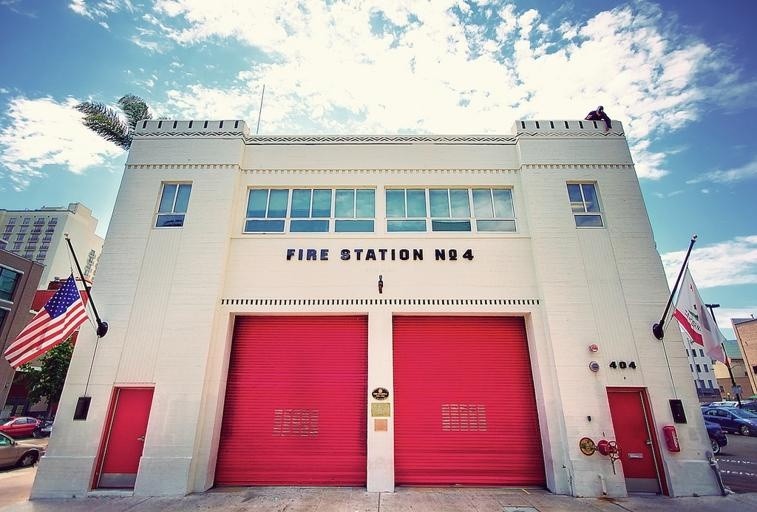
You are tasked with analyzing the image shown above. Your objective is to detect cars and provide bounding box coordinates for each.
[33,420,53,438]
[0,415,43,438]
[0,432,44,471]
[703,400,757,436]
[705,420,727,454]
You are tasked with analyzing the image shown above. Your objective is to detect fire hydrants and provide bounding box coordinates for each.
[580,437,623,460]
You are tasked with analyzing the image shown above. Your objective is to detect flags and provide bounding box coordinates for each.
[668,264,727,366]
[3,271,89,370]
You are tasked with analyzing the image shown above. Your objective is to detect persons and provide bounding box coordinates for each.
[584,106,611,131]
